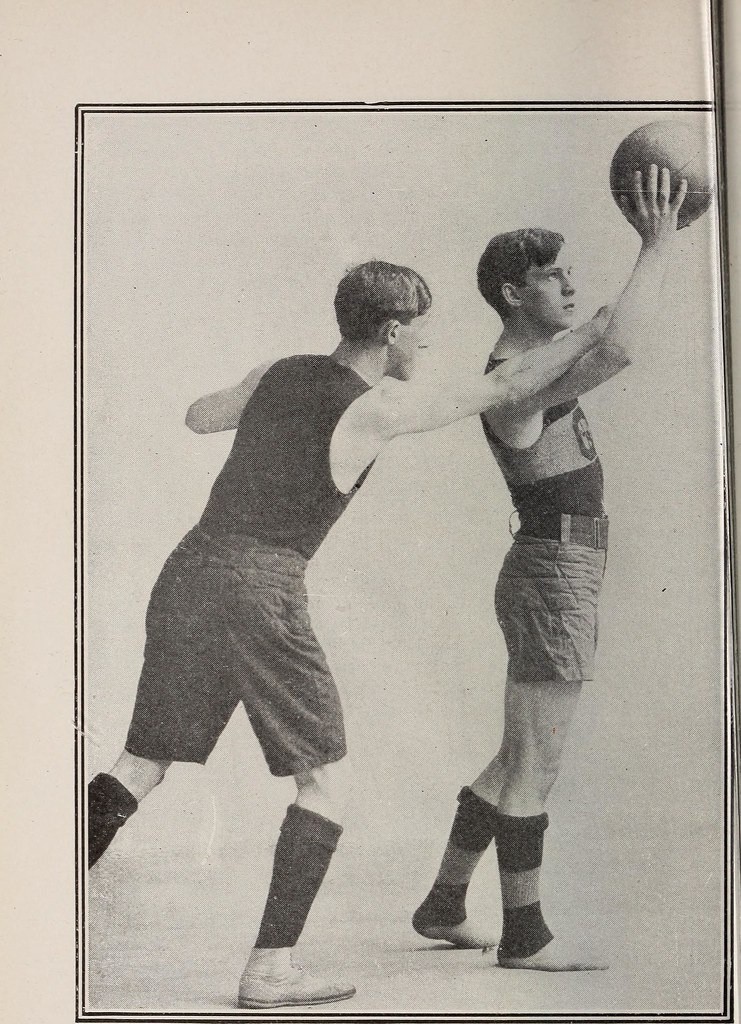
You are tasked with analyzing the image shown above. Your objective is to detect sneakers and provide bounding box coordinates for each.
[239,953,356,1009]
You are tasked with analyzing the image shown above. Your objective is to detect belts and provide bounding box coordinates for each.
[514,512,609,549]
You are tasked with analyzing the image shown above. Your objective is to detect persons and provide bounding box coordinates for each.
[412,164,688,974]
[85,261,618,1007]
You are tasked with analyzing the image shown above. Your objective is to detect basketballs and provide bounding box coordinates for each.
[610,118,712,229]
